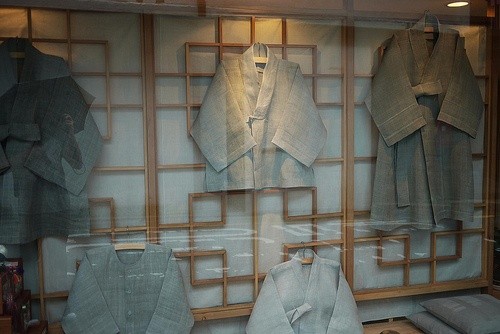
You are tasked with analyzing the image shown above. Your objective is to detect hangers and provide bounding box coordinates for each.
[114,225,145,250]
[8,35,25,59]
[424,8,436,33]
[253,41,267,63]
[299,240,314,265]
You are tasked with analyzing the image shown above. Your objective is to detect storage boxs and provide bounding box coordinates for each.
[0,315,14,334]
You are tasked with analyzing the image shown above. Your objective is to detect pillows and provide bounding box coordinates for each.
[405,310,462,334]
[422,293,500,334]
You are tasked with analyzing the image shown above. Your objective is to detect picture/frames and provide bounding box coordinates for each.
[10,288,33,334]
[6,257,24,291]
[25,320,49,334]
